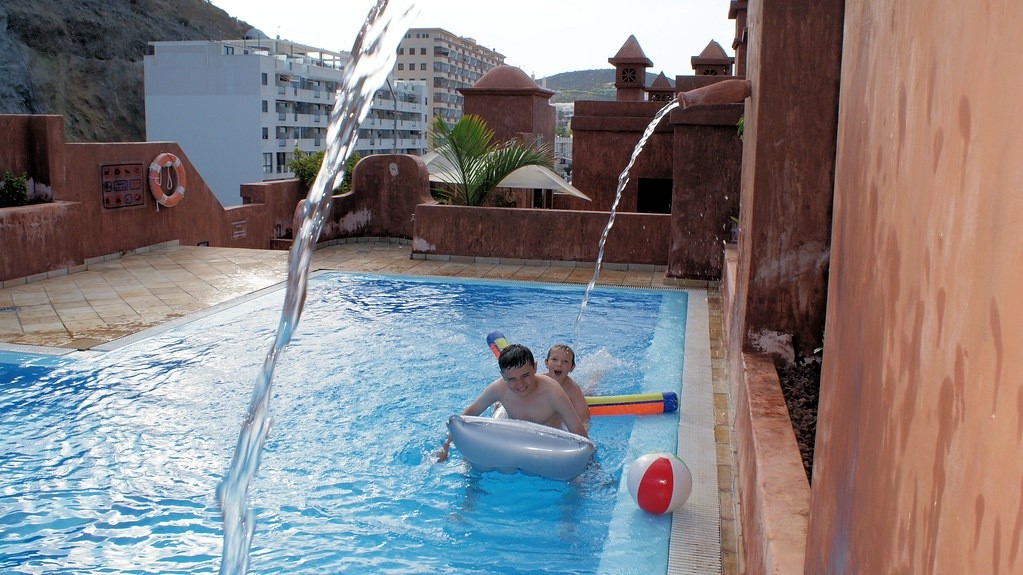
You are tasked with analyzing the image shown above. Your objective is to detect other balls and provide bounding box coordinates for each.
[627,449,693,516]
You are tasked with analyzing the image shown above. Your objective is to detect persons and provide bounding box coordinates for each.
[435,343,590,462]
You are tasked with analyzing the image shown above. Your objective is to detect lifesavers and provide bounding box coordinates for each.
[149,152,187,209]
[486,332,679,414]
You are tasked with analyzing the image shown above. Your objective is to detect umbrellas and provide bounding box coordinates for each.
[419,142,593,206]
